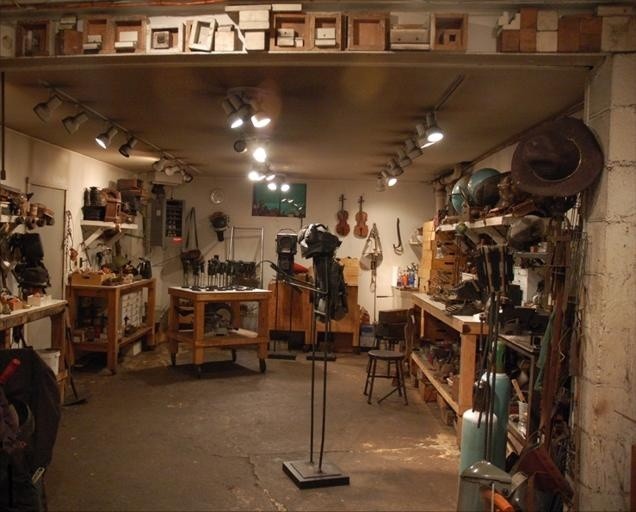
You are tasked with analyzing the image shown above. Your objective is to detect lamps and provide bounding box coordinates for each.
[33,79,202,182]
[223,94,290,192]
[377,73,465,192]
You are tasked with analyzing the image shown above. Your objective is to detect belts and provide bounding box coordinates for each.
[549,215,569,447]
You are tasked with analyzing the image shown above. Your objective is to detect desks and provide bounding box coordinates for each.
[66,279,156,374]
[169,286,272,378]
[412,292,489,446]
[0,300,68,410]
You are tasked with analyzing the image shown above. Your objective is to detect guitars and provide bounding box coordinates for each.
[354,196,368,237]
[336,195,349,236]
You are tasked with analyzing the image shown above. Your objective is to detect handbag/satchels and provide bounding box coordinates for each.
[180,249,205,265]
[0,348,61,474]
[509,443,573,503]
[361,253,383,269]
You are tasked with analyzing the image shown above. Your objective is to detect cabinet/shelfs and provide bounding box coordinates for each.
[392,287,416,310]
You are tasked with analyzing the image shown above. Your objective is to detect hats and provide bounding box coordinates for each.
[511,118,603,197]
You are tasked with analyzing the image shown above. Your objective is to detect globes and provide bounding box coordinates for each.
[447,168,501,216]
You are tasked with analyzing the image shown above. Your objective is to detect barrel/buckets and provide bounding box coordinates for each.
[37,347,61,376]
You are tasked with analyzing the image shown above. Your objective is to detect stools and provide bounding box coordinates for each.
[363,350,408,405]
